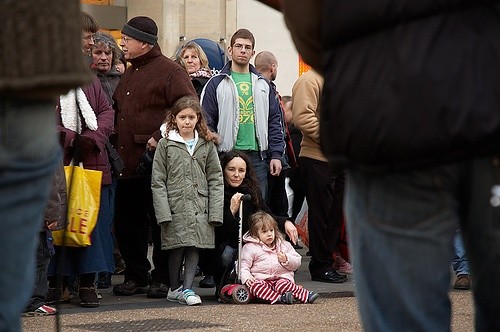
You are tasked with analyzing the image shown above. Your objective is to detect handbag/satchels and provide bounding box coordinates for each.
[108,146,127,175]
[51,158,103,247]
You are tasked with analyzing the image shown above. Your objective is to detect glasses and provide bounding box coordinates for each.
[121,35,133,40]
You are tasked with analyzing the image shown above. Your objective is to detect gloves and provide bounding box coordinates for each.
[72,132,100,153]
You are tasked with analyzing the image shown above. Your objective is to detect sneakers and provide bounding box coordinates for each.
[113,280,150,296]
[166,284,183,303]
[178,288,202,306]
[148,282,170,299]
[20,304,56,317]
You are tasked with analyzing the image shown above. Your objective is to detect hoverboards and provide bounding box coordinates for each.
[215,193,256,306]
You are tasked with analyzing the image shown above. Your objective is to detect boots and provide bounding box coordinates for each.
[47,280,62,304]
[79,279,99,308]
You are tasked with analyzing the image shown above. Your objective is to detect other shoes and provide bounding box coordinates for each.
[339,262,353,274]
[199,275,214,288]
[308,291,319,304]
[215,290,231,304]
[311,270,347,283]
[281,292,293,304]
[453,275,471,290]
[114,263,127,275]
[98,274,111,289]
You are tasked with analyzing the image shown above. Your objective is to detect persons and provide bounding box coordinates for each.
[0,0,90,332]
[258,1,500,332]
[171,41,214,288]
[150,97,224,306]
[200,27,285,197]
[54,14,125,308]
[239,210,319,307]
[254,52,353,282]
[451,234,471,290]
[110,15,198,299]
[211,151,270,294]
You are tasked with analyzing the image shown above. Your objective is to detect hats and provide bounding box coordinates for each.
[121,15,158,45]
